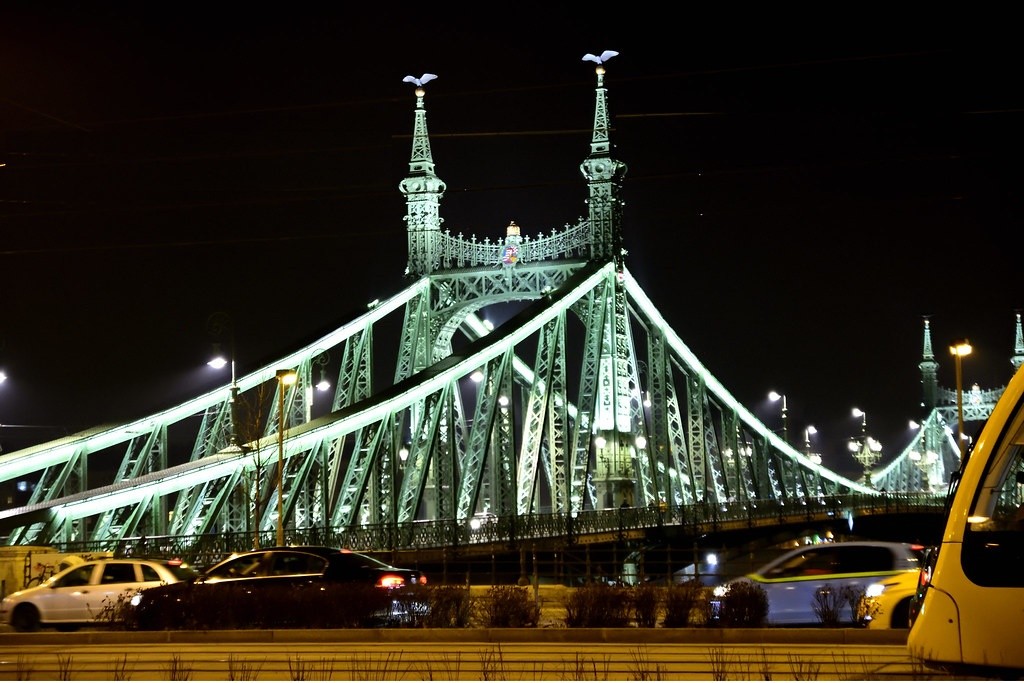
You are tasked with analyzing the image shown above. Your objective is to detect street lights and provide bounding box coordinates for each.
[805,425,817,460]
[275,369,299,548]
[846,408,882,489]
[208,356,242,445]
[399,444,408,477]
[949,342,973,462]
[768,391,788,442]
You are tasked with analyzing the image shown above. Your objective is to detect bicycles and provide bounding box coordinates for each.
[25,562,56,589]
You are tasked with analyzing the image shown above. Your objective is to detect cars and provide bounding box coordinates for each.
[907,363,1024,671]
[0,558,199,632]
[129,545,427,628]
[146,559,201,583]
[710,540,928,630]
